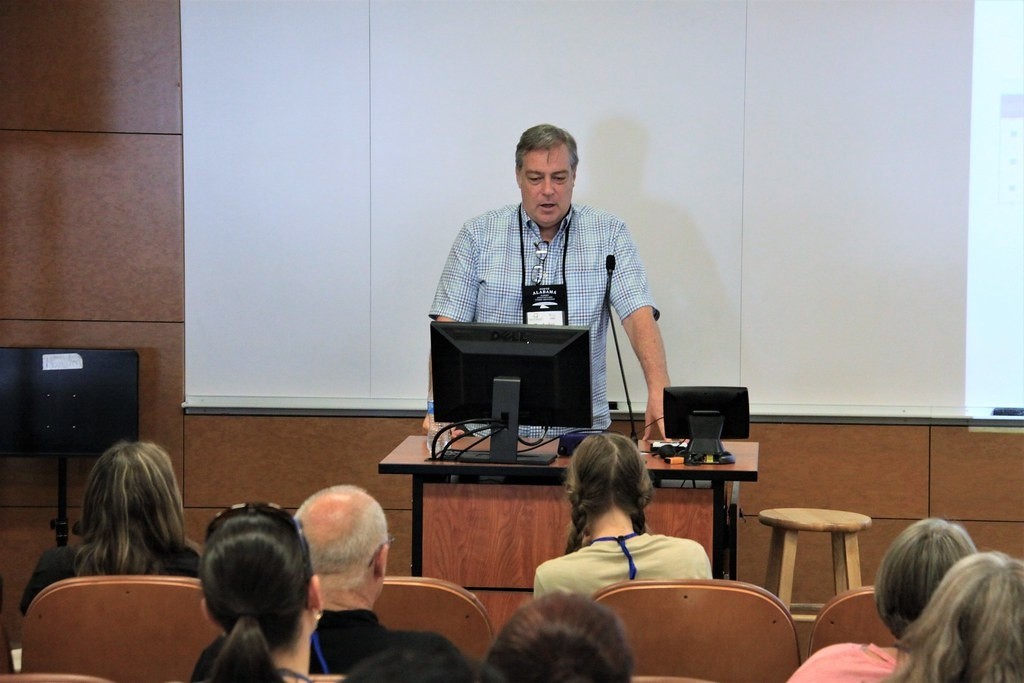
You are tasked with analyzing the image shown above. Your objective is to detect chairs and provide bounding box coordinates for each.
[376,577,491,662]
[21,576,219,683]
[809,588,892,659]
[587,579,801,683]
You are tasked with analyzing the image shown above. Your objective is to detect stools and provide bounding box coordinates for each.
[758,508,871,626]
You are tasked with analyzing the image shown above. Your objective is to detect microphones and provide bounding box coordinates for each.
[604,254,640,453]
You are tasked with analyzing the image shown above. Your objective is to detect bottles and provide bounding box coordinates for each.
[427,387,451,453]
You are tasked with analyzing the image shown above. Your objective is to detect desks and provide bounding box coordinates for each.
[377,435,760,624]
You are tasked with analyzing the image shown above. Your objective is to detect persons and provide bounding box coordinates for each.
[424,123,688,444]
[21,441,200,616]
[479,589,634,683]
[786,518,976,683]
[533,434,713,600]
[294,484,416,673]
[885,551,1024,683]
[339,634,472,683]
[191,504,344,683]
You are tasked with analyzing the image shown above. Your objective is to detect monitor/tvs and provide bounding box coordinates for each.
[430,321,594,466]
[664,387,750,464]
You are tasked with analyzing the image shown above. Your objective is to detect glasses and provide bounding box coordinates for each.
[530,240,549,288]
[204,501,300,542]
